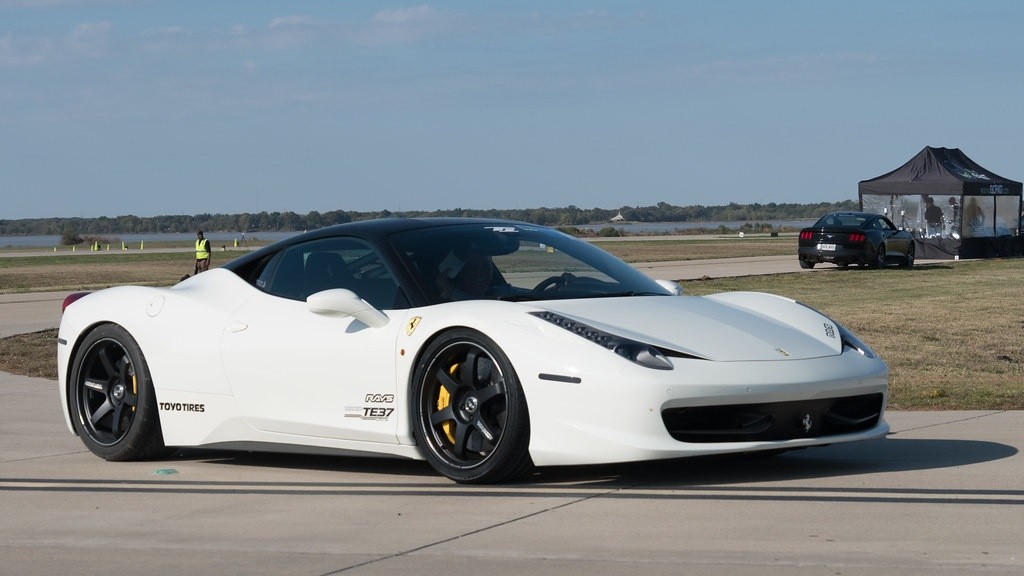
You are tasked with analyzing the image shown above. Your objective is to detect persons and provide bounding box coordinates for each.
[925,197,946,238]
[948,197,960,235]
[964,197,985,237]
[453,247,572,300]
[194,230,211,275]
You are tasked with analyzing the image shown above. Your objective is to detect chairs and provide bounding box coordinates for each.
[306,253,363,303]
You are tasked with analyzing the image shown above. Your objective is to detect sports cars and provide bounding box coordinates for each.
[56,216,890,484]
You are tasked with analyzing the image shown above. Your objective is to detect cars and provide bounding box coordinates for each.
[798,212,915,270]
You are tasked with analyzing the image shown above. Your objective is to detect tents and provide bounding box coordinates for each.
[858,145,1024,259]
